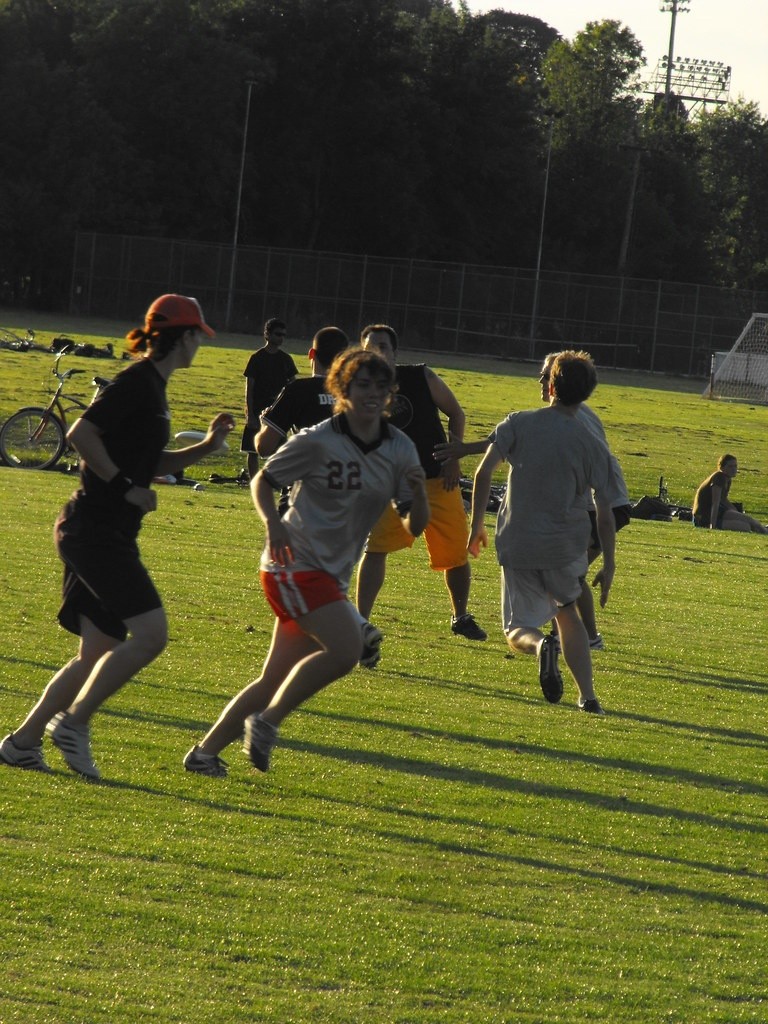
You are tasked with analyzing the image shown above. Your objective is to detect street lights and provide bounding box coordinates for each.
[614,142,659,270]
[527,104,567,358]
[223,72,260,329]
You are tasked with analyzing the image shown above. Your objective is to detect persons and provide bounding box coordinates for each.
[0,294,237,776]
[240,319,299,479]
[360,324,487,641]
[253,326,382,669]
[183,346,430,778]
[466,349,620,717]
[432,354,631,646]
[692,454,768,533]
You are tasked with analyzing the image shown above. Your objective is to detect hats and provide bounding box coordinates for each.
[145,294,216,337]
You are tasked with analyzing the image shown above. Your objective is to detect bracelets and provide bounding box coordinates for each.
[111,471,136,495]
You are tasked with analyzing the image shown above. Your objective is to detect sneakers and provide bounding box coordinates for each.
[0,733,52,772]
[45,711,100,778]
[549,631,562,650]
[589,632,603,649]
[360,622,382,669]
[244,713,277,773]
[578,697,606,715]
[450,615,488,641]
[183,745,229,778]
[538,636,563,703]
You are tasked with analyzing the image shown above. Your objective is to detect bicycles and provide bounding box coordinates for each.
[0,344,114,470]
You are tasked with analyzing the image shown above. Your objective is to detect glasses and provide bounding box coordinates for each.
[540,372,550,381]
[270,332,285,337]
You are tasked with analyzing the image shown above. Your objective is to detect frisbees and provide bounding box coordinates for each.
[174,432,229,455]
